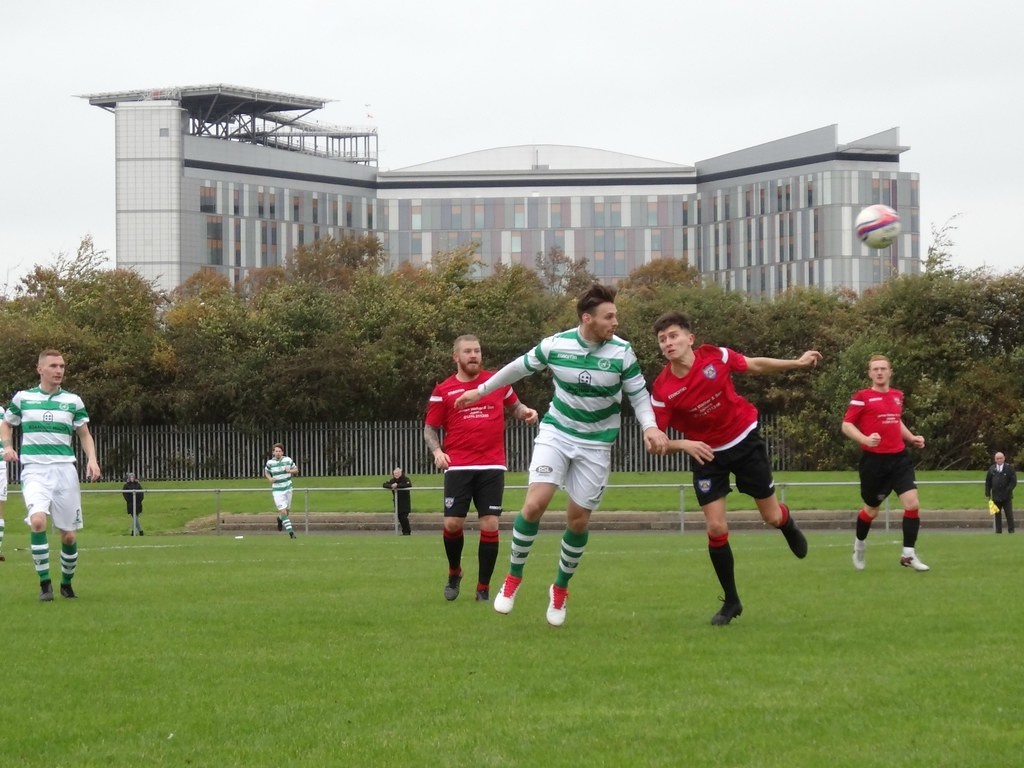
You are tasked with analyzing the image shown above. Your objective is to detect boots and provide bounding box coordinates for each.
[139,530,143,537]
[131,530,134,536]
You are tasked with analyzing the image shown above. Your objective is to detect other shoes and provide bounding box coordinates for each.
[289,531,296,538]
[0,554,5,561]
[277,517,284,531]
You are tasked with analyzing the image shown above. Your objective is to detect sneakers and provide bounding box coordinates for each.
[899,553,929,571]
[39,578,54,601]
[781,517,808,557]
[546,583,570,626]
[853,540,866,570]
[475,586,488,600]
[494,574,523,614]
[712,595,742,625]
[60,584,77,598]
[444,567,464,601]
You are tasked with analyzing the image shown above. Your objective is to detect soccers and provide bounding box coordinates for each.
[854,204,904,250]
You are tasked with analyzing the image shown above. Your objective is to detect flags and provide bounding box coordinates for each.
[988,498,1000,517]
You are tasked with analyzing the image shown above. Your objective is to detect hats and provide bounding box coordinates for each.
[127,473,135,482]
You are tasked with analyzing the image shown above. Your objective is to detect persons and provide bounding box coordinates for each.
[384,467,413,535]
[985,451,1018,534]
[841,354,931,570]
[122,473,145,535]
[264,443,299,539]
[642,313,824,627]
[0,350,100,601]
[454,289,669,625]
[423,333,539,601]
[0,406,12,565]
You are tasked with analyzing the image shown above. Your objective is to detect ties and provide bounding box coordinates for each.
[998,464,1001,472]
